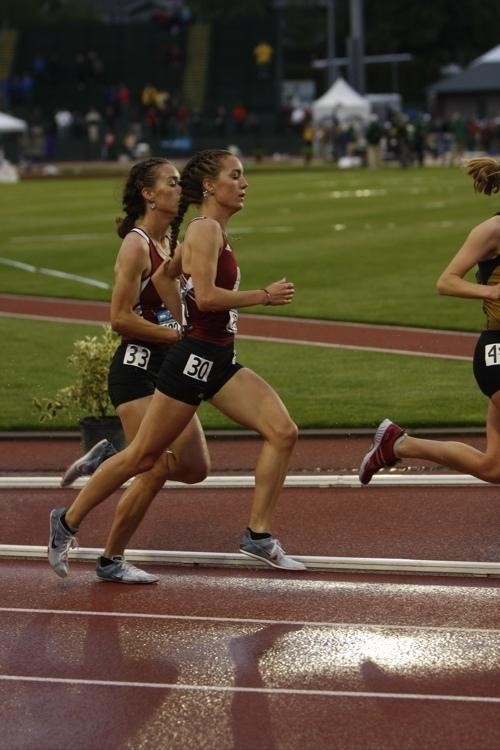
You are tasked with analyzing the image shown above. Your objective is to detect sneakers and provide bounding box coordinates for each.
[238,531,307,572]
[96,555,159,584]
[61,440,117,488]
[358,417,404,485]
[47,508,76,578]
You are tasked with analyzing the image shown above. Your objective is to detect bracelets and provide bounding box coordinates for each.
[261,285,273,308]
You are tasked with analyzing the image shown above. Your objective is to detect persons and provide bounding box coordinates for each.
[56,158,213,585]
[48,150,309,578]
[22,82,499,176]
[358,157,499,488]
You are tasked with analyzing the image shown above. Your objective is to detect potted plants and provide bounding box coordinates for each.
[29,324,122,453]
